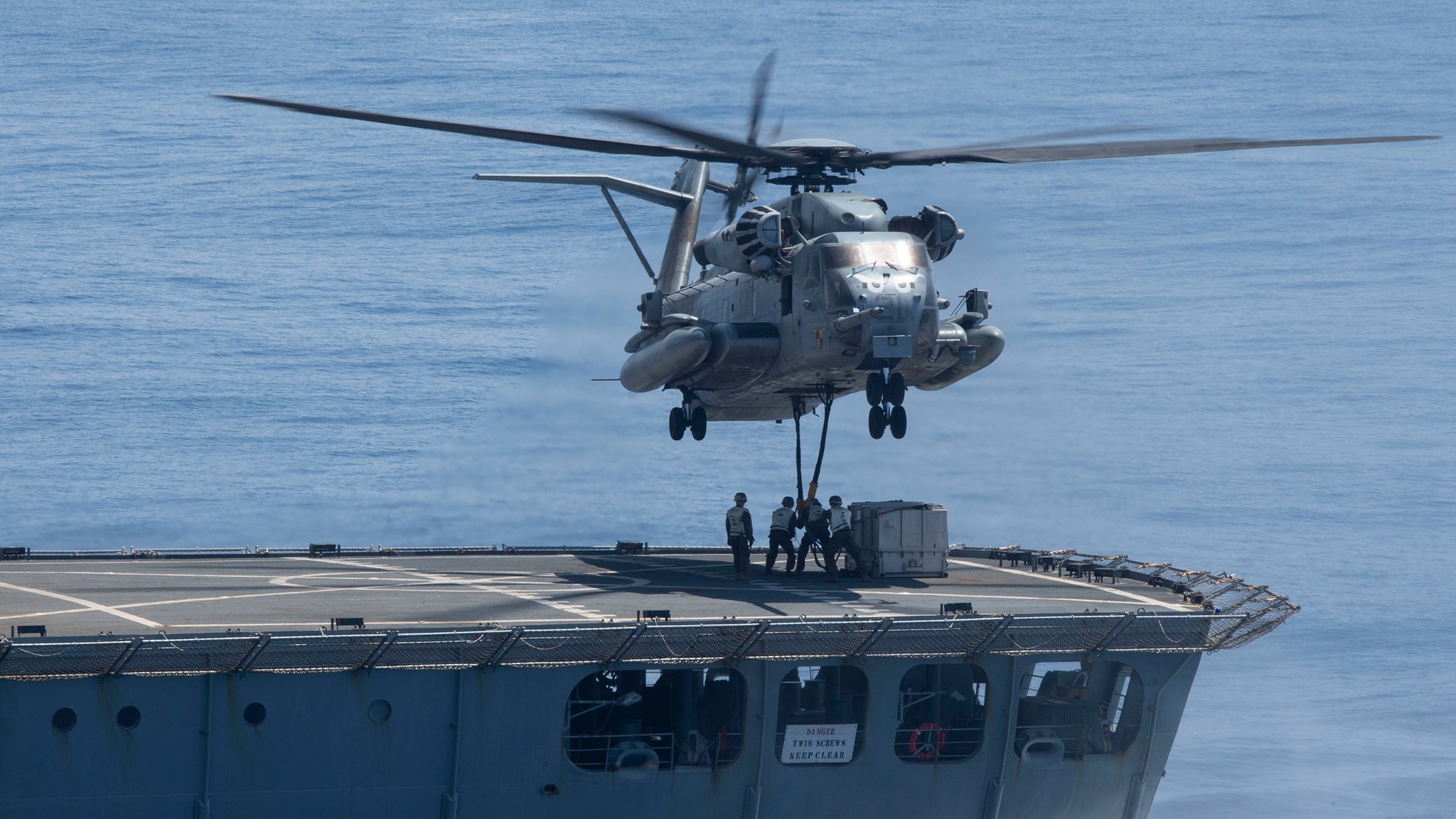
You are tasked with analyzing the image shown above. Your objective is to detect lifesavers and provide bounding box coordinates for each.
[909,722,946,761]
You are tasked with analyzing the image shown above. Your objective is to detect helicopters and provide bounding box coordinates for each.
[212,48,1440,443]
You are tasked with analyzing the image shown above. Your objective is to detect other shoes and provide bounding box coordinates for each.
[736,572,741,581]
[824,573,839,582]
[764,568,775,575]
[786,569,797,577]
[740,572,747,581]
[792,568,802,575]
[860,571,874,582]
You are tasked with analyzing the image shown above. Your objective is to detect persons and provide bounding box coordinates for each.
[794,497,838,577]
[819,496,873,583]
[764,497,797,576]
[726,492,755,582]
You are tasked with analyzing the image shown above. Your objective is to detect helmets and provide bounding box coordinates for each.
[781,497,794,505]
[734,492,747,501]
[812,498,822,506]
[829,496,842,505]
